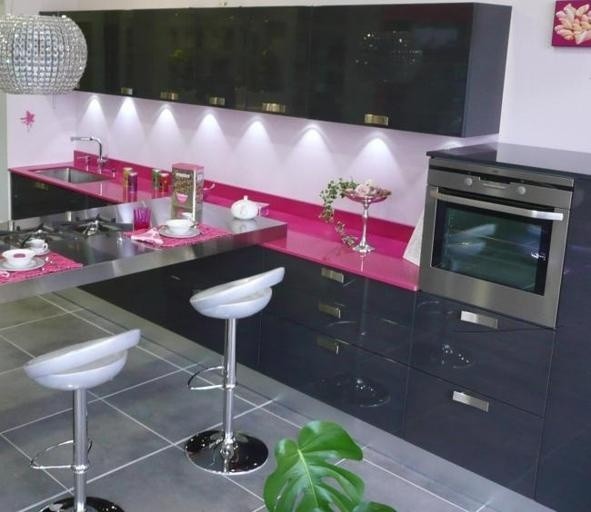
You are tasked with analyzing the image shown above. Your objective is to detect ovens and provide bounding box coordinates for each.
[420,157,574,332]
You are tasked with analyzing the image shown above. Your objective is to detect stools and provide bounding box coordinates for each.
[23,329,141,512]
[184,267,286,476]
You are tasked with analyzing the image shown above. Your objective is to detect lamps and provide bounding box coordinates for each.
[1,12,88,96]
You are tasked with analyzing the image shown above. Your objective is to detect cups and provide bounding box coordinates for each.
[31,239,48,254]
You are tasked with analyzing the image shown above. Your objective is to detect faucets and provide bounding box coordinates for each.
[69,136,103,160]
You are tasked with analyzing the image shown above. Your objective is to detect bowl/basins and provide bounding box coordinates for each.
[166,219,194,234]
[1,249,36,267]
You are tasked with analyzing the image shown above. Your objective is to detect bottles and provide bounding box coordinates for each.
[160,173,168,192]
[123,167,133,185]
[152,169,161,188]
[128,172,138,191]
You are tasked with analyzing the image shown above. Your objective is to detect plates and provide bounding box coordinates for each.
[28,247,49,256]
[159,227,201,238]
[0,256,45,272]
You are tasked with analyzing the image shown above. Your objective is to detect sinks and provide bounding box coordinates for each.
[29,166,112,185]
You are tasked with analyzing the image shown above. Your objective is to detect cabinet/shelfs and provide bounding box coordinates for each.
[133,9,181,101]
[12,173,108,218]
[78,245,254,369]
[340,3,512,137]
[256,248,556,497]
[40,11,89,93]
[89,11,133,97]
[239,7,339,124]
[182,9,239,110]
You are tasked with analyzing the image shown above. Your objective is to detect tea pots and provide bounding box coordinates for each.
[231,194,258,219]
